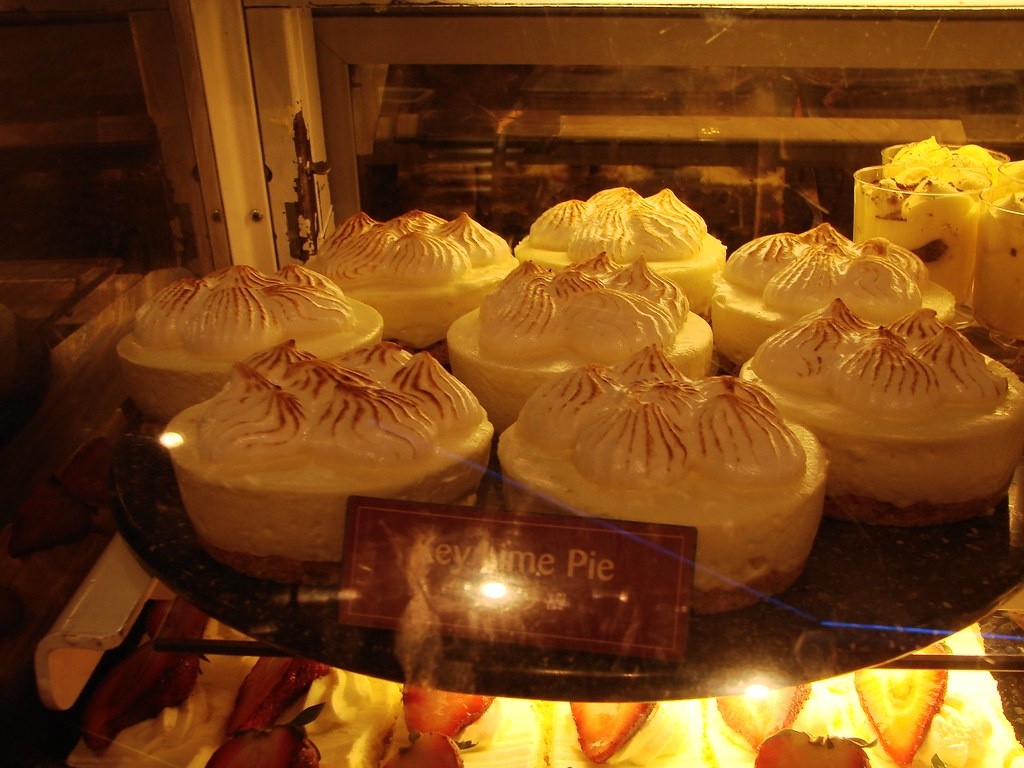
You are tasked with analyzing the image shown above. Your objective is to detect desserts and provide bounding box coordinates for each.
[113,132,1024,615]
[77,579,1019,766]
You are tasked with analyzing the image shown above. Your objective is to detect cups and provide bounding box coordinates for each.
[852,144,1024,341]
